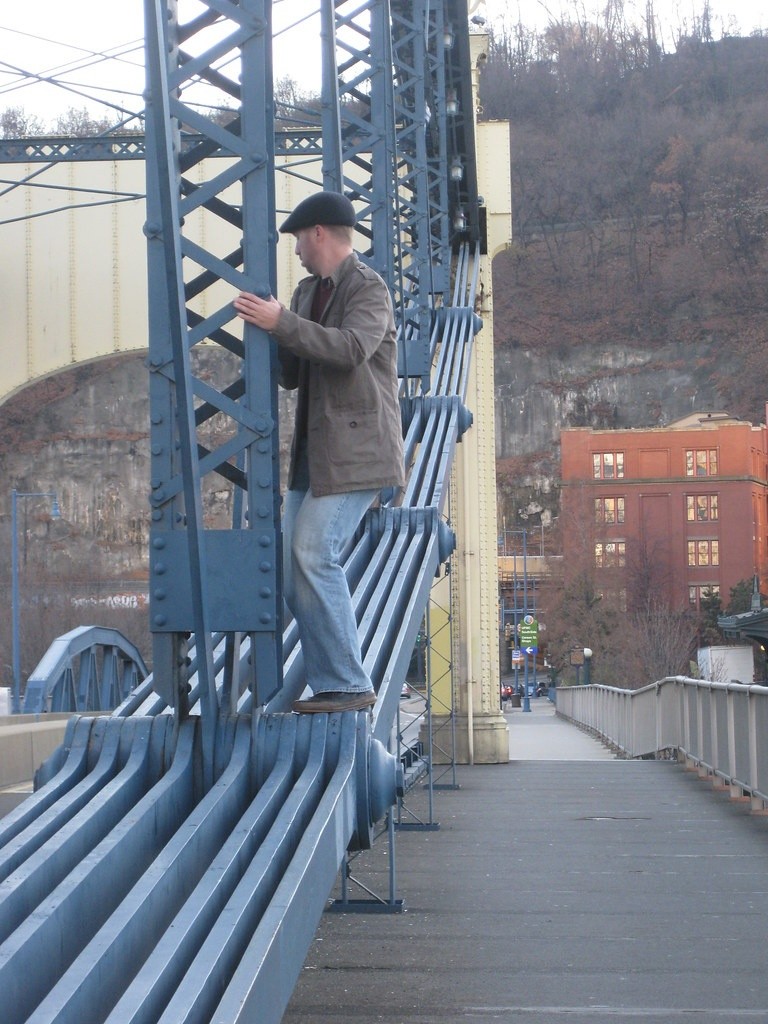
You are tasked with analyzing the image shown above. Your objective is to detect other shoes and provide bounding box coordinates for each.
[293,688,378,712]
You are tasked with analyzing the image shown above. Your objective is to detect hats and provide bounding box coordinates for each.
[279,192,358,233]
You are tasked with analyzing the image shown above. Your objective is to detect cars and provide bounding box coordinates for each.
[501,681,547,701]
[400,683,411,699]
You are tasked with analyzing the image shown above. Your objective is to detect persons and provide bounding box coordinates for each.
[233,193,406,712]
[519,684,525,699]
